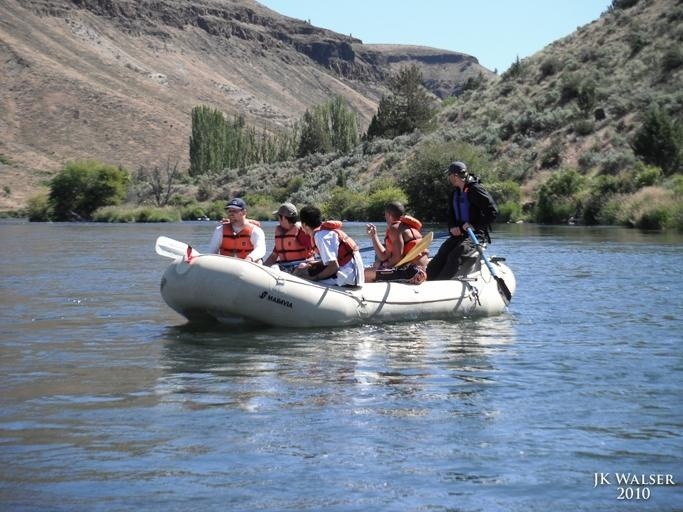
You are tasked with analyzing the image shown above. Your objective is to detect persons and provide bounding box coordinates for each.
[297,206,365,288]
[262,202,319,274]
[362,201,430,282]
[208,198,266,265]
[427,161,498,280]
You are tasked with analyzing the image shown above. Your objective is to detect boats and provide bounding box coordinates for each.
[162,253,513,325]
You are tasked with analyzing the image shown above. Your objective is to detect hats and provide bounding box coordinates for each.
[272,203,299,218]
[223,198,245,210]
[442,161,467,174]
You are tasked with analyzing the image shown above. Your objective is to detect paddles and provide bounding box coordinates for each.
[394,232,433,268]
[155,236,203,259]
[468,227,511,307]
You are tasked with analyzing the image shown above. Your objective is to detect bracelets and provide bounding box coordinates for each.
[316,274,320,280]
